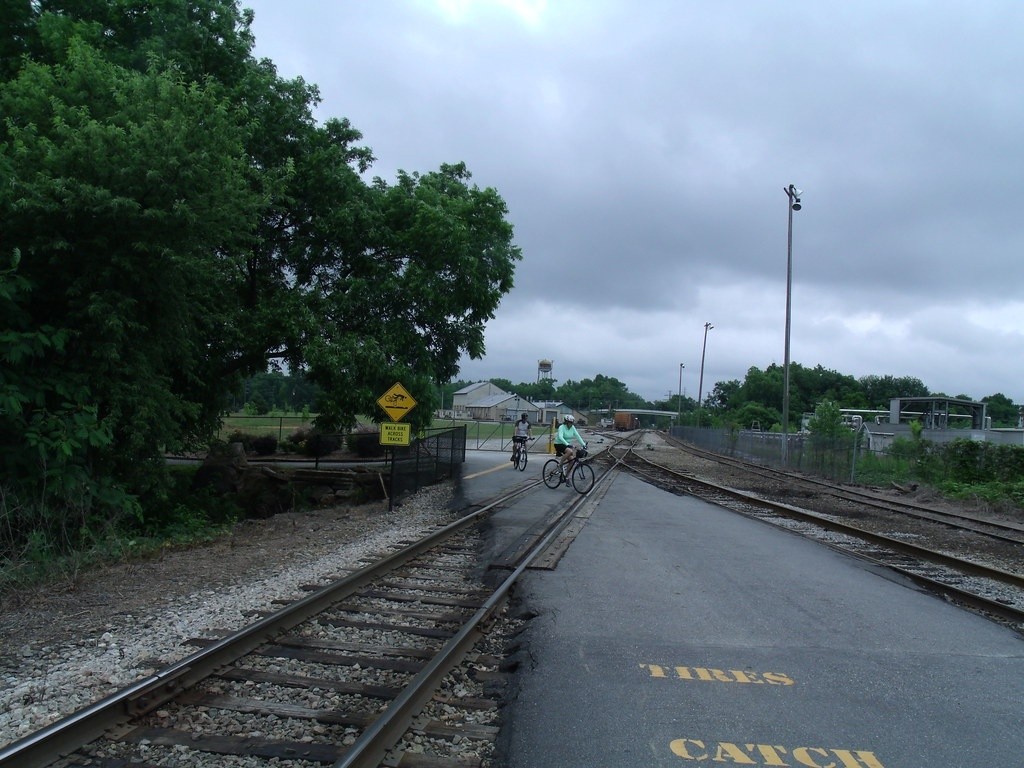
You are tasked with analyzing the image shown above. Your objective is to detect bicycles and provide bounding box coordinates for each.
[542,442,595,495]
[512,437,536,471]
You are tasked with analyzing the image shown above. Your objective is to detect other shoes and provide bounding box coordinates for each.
[558,464,564,474]
[565,479,572,487]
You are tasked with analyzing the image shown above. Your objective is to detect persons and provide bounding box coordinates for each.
[554,415,587,487]
[511,413,532,459]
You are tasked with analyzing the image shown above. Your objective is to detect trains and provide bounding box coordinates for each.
[614,413,640,431]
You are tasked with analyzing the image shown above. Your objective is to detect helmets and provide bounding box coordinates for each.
[564,414,576,423]
[521,413,528,419]
